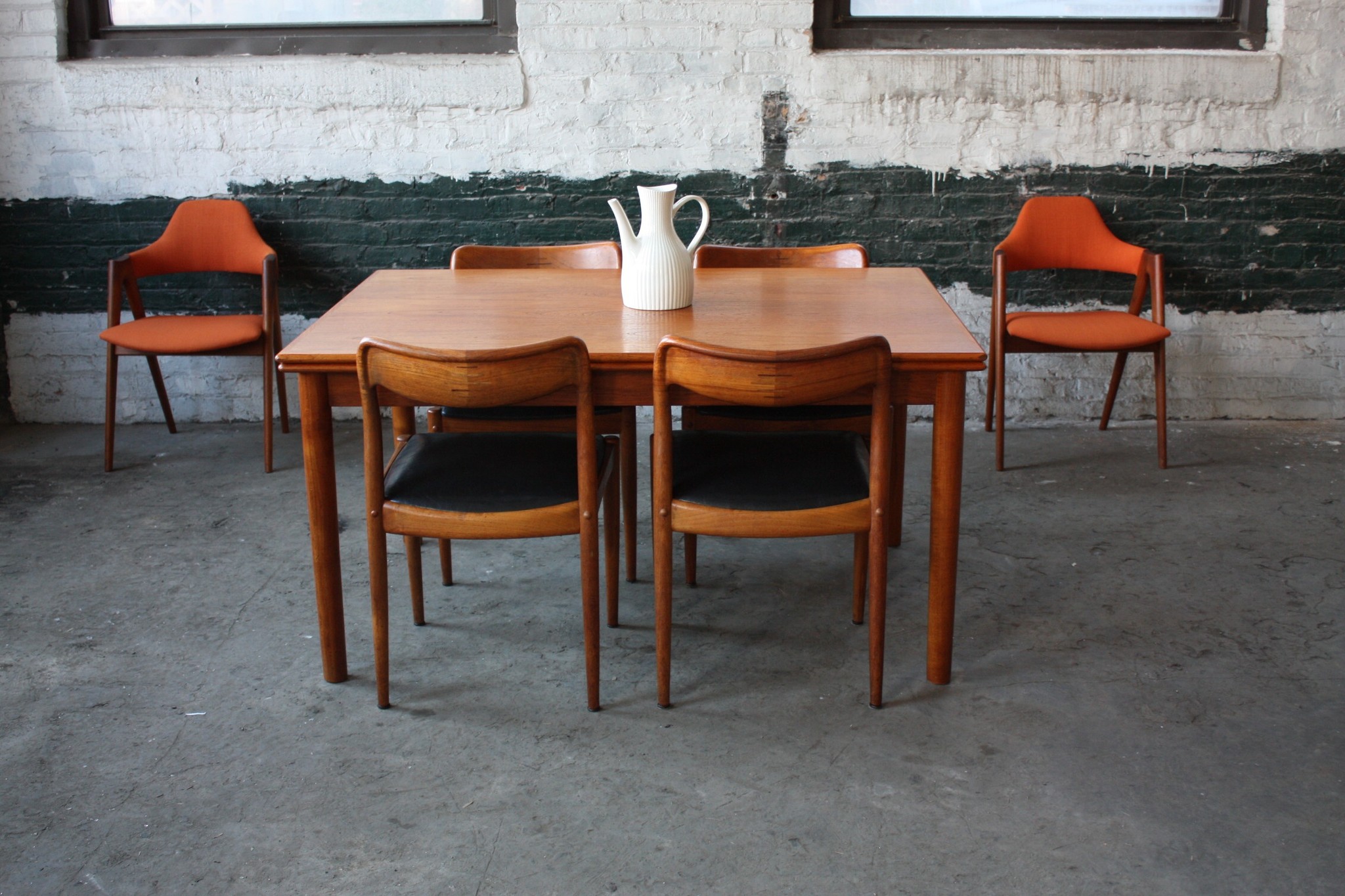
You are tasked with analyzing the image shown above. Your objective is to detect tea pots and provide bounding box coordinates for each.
[607,183,711,311]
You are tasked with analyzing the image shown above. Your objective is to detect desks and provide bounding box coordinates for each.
[273,268,986,685]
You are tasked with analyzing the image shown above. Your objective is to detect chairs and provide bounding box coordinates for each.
[356,241,891,712]
[99,200,289,473]
[986,196,1170,470]
